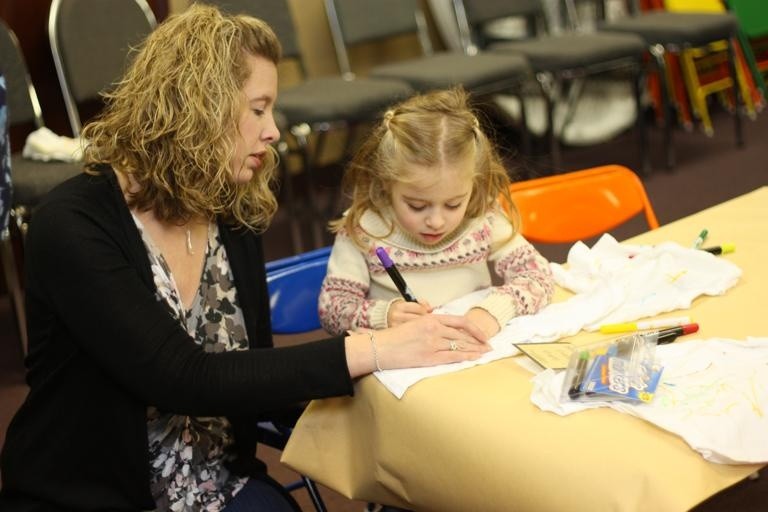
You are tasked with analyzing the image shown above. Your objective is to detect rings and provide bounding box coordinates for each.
[448,339,457,352]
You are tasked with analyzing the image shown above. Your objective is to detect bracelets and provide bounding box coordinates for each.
[367,332,381,371]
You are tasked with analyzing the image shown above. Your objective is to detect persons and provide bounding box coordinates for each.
[0,3,493,512]
[315,82,556,338]
[0,55,26,390]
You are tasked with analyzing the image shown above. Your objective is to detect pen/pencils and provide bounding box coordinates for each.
[692,229,737,255]
[569,315,699,398]
[376,246,418,303]
